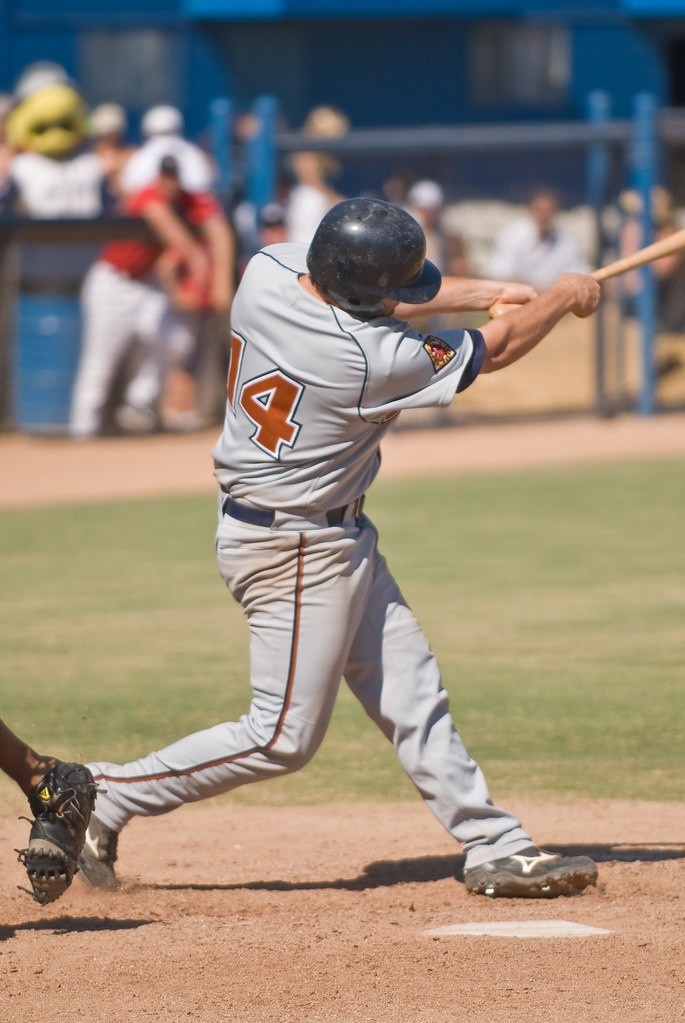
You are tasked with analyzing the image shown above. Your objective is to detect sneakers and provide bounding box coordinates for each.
[464,846,600,899]
[74,811,118,892]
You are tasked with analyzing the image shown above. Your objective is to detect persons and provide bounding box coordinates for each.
[0,60,236,441]
[491,186,684,332]
[282,109,480,290]
[1,720,97,905]
[78,197,605,898]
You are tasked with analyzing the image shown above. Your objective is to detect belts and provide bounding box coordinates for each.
[222,498,365,529]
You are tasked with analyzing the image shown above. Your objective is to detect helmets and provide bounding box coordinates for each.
[305,197,442,323]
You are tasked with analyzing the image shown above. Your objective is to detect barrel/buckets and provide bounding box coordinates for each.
[13,293,83,437]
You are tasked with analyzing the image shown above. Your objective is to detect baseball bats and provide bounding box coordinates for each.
[489,229,685,317]
